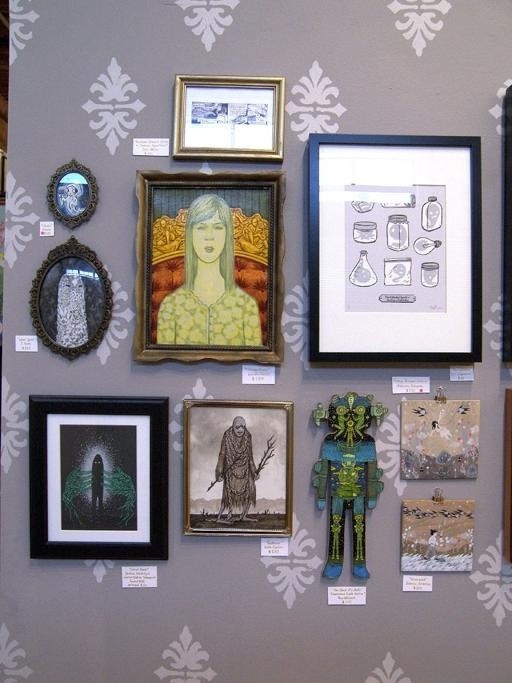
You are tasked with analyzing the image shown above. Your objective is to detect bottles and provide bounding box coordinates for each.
[352,221,378,244]
[420,195,442,232]
[347,249,379,289]
[420,262,440,289]
[386,214,410,253]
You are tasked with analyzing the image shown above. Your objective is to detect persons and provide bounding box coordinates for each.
[215,414,262,525]
[157,192,265,348]
[424,528,443,561]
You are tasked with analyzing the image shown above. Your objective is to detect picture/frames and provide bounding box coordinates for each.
[133,169,284,365]
[399,400,480,480]
[29,396,171,559]
[182,399,293,536]
[173,76,285,160]
[29,235,116,362]
[47,159,99,231]
[401,499,474,571]
[307,134,483,362]
